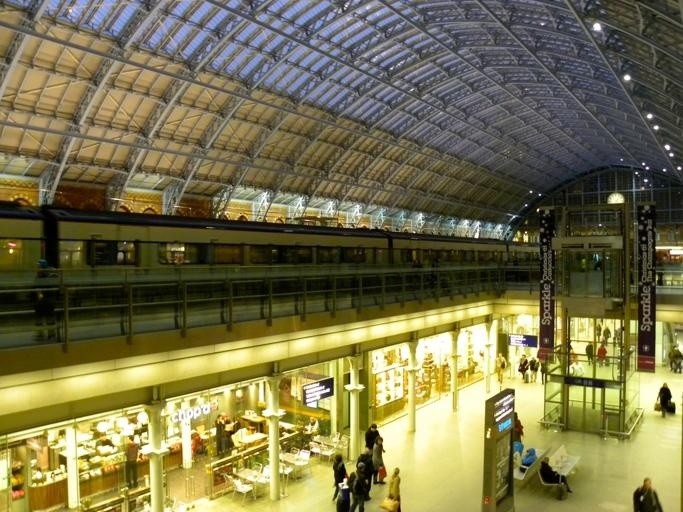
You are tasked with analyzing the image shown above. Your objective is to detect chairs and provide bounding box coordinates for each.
[221,430,349,505]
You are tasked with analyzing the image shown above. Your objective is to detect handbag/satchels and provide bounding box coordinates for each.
[379,494,400,511]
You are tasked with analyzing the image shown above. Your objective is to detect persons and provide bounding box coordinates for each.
[411,258,439,292]
[332,424,401,512]
[27,259,57,341]
[513,448,537,473]
[514,413,524,442]
[96,432,114,449]
[307,417,319,432]
[540,457,573,493]
[633,478,662,512]
[125,435,139,490]
[668,346,683,373]
[656,383,672,418]
[495,324,611,384]
[215,414,233,458]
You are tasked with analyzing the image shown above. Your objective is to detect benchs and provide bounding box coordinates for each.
[512,446,552,486]
[536,444,581,486]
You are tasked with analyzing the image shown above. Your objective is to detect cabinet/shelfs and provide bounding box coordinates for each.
[371,362,406,420]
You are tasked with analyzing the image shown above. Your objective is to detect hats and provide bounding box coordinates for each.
[358,462,367,469]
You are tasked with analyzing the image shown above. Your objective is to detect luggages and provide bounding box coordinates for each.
[525,372,530,384]
[667,399,677,414]
[555,484,569,500]
